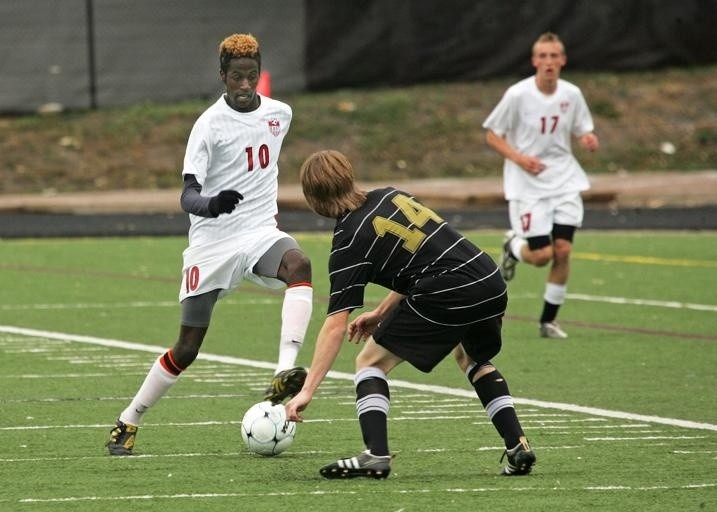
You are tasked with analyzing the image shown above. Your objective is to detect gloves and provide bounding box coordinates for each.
[208,189,243,219]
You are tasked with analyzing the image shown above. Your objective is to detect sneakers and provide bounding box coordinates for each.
[107,416,139,457]
[497,435,537,477]
[318,448,391,481]
[262,366,307,407]
[498,230,519,283]
[536,319,569,339]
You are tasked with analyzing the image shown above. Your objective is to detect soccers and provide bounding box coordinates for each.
[240,401,296,456]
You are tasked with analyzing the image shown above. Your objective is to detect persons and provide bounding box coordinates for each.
[105,33,313,454]
[283,149,538,481]
[481,32,600,338]
